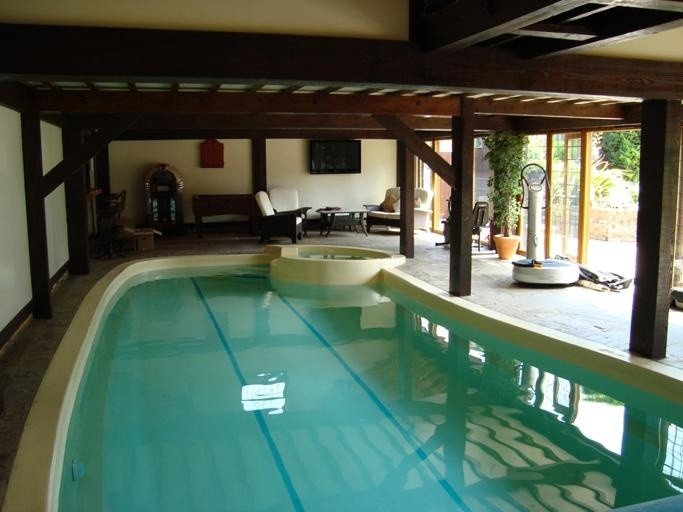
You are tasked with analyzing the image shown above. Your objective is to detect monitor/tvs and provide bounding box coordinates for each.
[309,140,361,174]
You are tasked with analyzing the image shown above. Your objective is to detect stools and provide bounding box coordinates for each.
[512,259,581,286]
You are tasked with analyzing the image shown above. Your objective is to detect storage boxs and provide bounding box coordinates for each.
[124,225,163,252]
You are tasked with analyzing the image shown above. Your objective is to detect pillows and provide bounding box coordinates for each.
[380,193,400,212]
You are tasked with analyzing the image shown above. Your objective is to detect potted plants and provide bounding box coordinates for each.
[482,131,533,261]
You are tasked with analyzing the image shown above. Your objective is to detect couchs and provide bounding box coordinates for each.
[363,187,434,233]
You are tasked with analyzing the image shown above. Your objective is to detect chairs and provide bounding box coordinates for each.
[471,201,490,252]
[255,187,313,244]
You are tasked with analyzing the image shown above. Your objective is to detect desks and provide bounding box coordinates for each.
[191,194,257,240]
